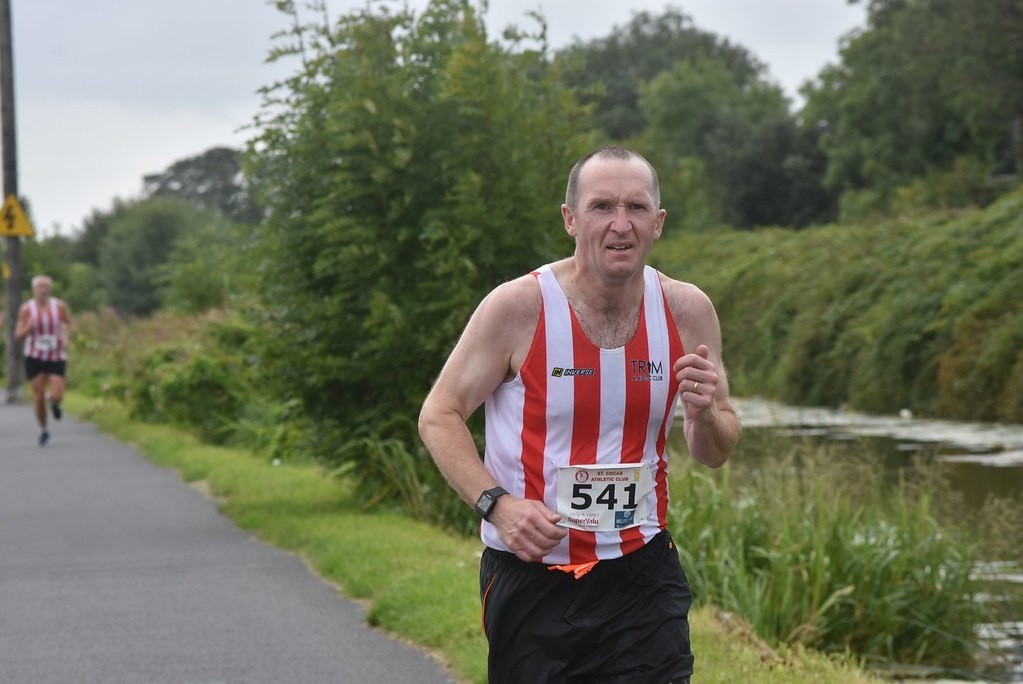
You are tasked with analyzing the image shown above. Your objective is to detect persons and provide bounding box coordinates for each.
[418,148,740,684]
[15,276,75,444]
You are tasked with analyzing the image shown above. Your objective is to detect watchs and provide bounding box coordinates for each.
[475,487,510,523]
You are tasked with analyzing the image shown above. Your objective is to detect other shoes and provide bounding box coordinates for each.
[39,432,50,446]
[51,406,61,418]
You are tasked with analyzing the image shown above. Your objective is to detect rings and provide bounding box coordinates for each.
[694,381,697,393]
